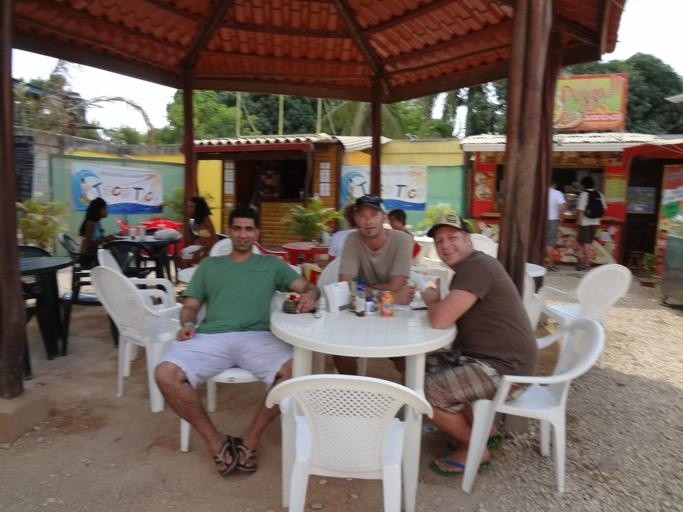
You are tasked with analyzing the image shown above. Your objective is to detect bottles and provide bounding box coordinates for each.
[380,291,394,318]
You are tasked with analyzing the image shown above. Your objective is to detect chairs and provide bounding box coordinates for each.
[59,272,120,356]
[175,266,302,454]
[97,248,185,321]
[317,256,341,297]
[471,233,498,258]
[90,266,183,412]
[254,240,288,263]
[59,233,97,290]
[102,240,165,291]
[532,263,632,369]
[265,374,434,512]
[18,245,52,257]
[116,218,138,235]
[461,315,606,494]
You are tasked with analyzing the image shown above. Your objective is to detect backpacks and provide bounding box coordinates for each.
[582,188,604,220]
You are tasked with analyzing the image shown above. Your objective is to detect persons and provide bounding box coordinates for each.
[249,174,281,215]
[176,197,218,271]
[421,212,539,477]
[317,204,358,268]
[333,194,415,378]
[545,180,567,271]
[153,206,321,478]
[78,197,117,267]
[573,175,608,272]
[388,209,415,247]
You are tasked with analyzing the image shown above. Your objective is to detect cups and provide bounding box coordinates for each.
[129,229,136,240]
[137,226,145,241]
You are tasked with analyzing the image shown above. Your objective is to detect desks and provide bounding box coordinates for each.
[280,241,330,266]
[18,257,75,379]
[111,235,182,291]
[270,294,456,511]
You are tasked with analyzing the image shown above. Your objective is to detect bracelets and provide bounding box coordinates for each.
[184,321,194,329]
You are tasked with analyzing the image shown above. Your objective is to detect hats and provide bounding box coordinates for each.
[343,193,384,226]
[426,213,470,236]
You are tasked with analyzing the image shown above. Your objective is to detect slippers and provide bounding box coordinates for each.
[429,452,494,477]
[446,432,505,449]
[232,437,258,473]
[211,434,238,476]
